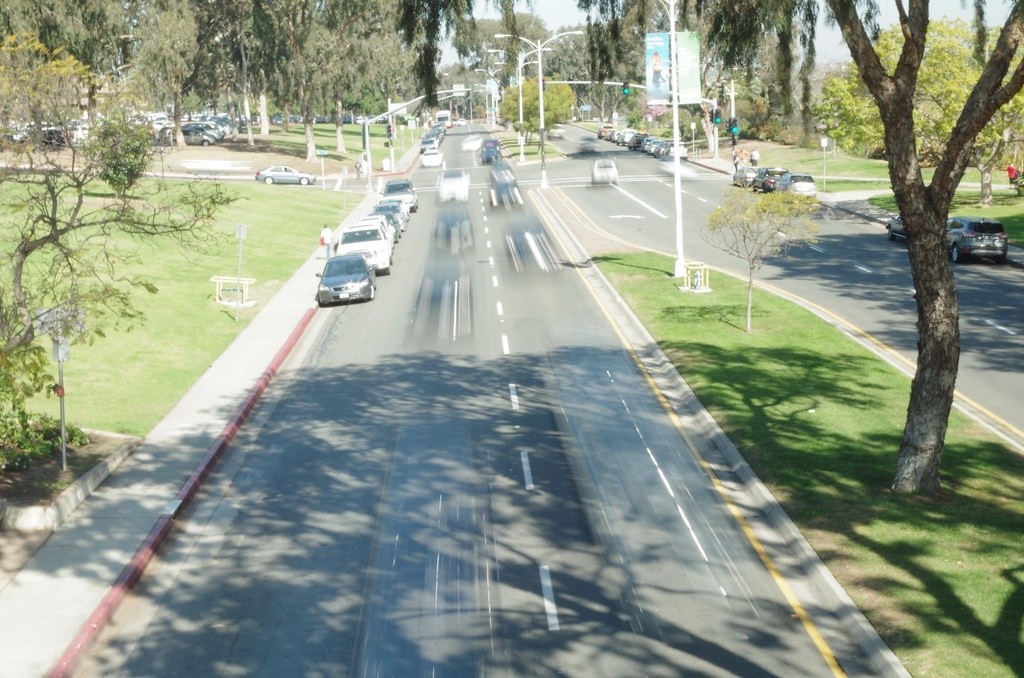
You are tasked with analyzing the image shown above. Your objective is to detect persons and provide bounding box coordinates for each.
[750,149,760,166]
[738,147,749,166]
[1007,163,1016,189]
[354,161,361,180]
[429,120,432,128]
[320,224,333,258]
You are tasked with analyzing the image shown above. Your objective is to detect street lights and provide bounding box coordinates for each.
[493,31,584,189]
[474,47,553,162]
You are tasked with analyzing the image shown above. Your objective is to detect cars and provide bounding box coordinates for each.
[481,139,501,165]
[733,166,765,189]
[315,255,377,307]
[885,216,907,242]
[597,125,689,162]
[417,110,467,168]
[592,158,618,184]
[256,166,316,186]
[333,177,418,276]
[11,111,389,147]
[488,171,525,209]
[775,173,817,199]
[437,166,470,203]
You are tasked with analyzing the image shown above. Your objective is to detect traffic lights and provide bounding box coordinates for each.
[623,81,629,94]
[731,117,739,134]
[709,109,714,123]
[715,108,722,124]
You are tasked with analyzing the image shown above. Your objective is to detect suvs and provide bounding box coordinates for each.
[752,167,788,193]
[945,216,1008,264]
